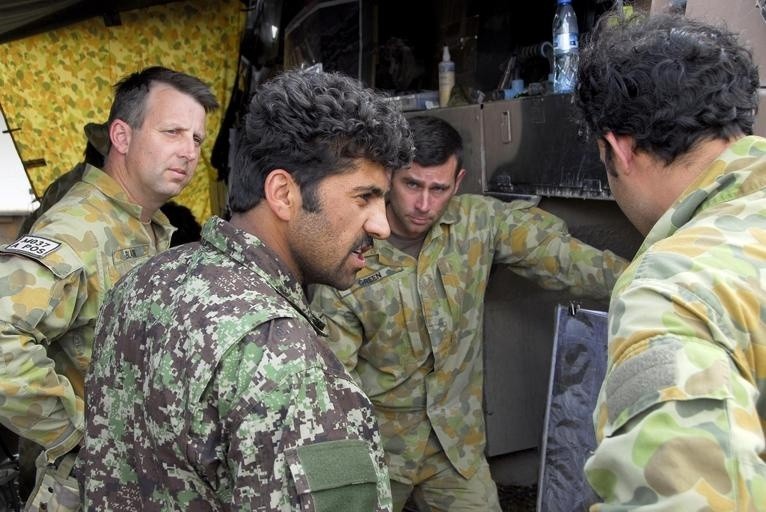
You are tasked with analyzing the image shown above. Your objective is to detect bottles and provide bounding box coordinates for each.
[550,0,581,95]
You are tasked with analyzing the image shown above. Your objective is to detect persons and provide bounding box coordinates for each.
[12,118,110,507]
[307,114,634,512]
[77,63,417,512]
[571,2,766,511]
[0,64,220,511]
